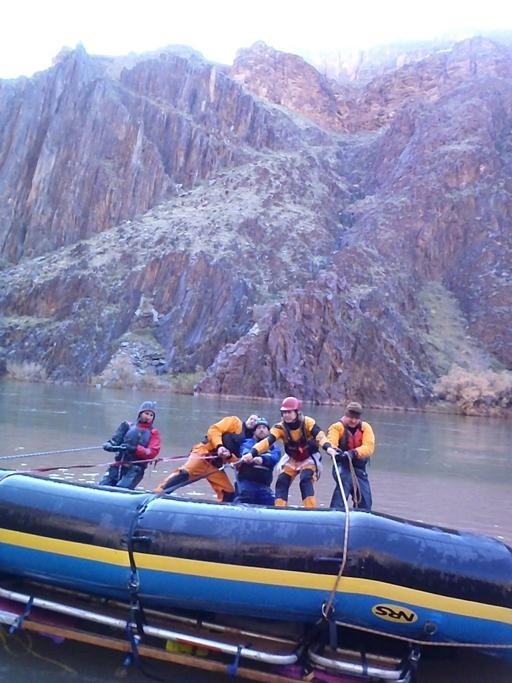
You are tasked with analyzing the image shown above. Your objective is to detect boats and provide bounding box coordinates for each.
[0,469,512,658]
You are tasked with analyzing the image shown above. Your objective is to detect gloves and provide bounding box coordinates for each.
[104,441,115,452]
[331,449,357,461]
[120,442,136,452]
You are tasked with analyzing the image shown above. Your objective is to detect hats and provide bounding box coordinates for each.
[253,418,270,430]
[345,402,363,418]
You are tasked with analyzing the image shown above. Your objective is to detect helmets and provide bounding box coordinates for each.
[137,401,155,419]
[280,397,300,411]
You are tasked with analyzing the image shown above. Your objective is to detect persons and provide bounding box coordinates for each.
[101,400,163,490]
[240,395,340,507]
[231,416,282,504]
[153,412,260,502]
[324,400,375,512]
